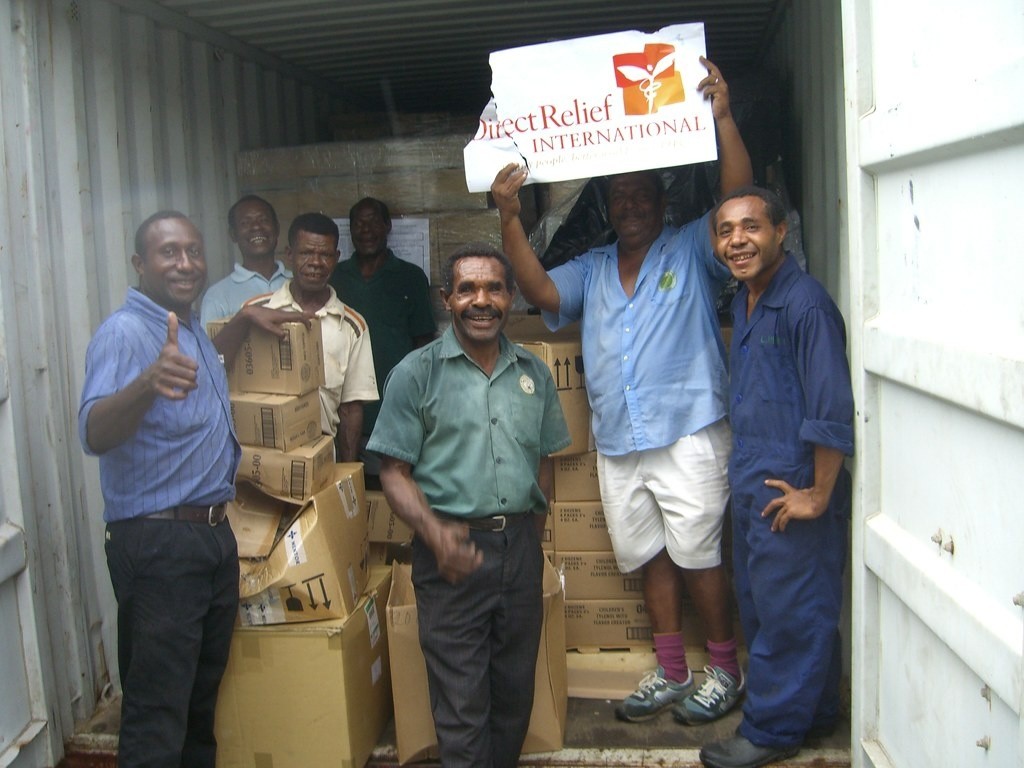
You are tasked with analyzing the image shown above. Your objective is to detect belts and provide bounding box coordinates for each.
[438,512,532,532]
[145,502,226,527]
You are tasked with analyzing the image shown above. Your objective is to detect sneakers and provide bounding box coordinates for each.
[615,663,695,723]
[671,664,745,726]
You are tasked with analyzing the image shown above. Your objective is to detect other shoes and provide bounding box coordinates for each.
[699,734,801,768]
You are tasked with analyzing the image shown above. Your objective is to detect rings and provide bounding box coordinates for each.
[714,77,719,86]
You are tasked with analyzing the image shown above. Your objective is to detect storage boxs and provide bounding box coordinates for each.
[213,110,742,768]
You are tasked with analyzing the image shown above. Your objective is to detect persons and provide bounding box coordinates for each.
[198,192,436,484]
[366,241,571,768]
[700,187,856,767]
[75,209,320,768]
[490,56,757,725]
